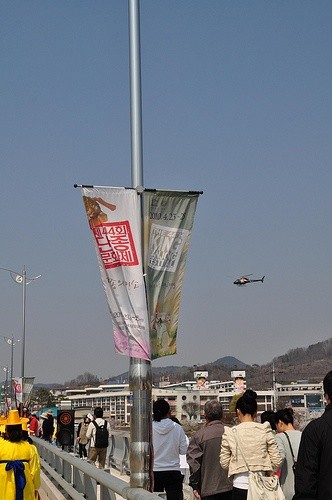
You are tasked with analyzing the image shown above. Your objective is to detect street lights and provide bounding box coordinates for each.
[0,332,22,410]
[0,264,44,418]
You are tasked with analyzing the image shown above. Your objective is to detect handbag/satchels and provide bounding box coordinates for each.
[247,471,285,500]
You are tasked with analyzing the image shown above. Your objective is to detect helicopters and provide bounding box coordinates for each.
[233,273,266,287]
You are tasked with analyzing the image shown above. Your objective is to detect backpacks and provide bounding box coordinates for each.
[92,420,109,448]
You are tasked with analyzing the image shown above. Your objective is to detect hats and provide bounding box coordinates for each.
[40,413,48,420]
[0,409,29,425]
[22,424,27,431]
[47,410,53,415]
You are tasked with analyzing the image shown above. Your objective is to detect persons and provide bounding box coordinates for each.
[22,407,58,444]
[186,390,302,500]
[151,398,189,500]
[0,410,40,500]
[293,370,332,500]
[77,407,112,470]
